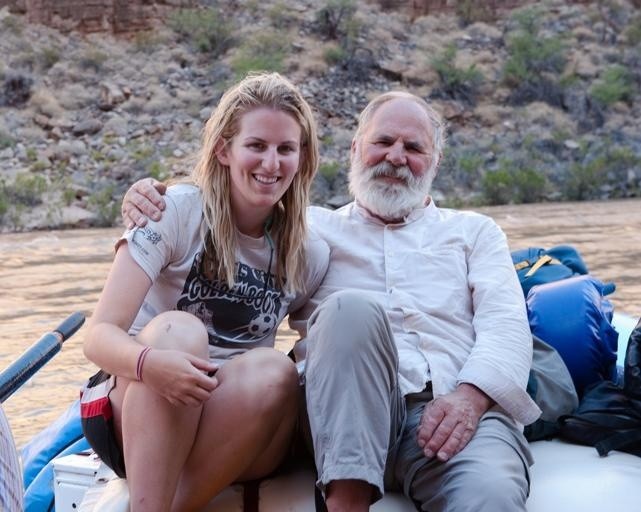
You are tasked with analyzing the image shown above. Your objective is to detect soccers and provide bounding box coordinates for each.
[249,313,275,337]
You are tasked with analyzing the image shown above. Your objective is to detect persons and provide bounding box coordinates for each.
[122,89,541,512]
[79,74,332,512]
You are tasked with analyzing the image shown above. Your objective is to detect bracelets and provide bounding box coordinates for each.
[135,347,150,381]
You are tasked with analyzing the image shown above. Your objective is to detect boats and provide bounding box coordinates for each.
[22,301,641,511]
